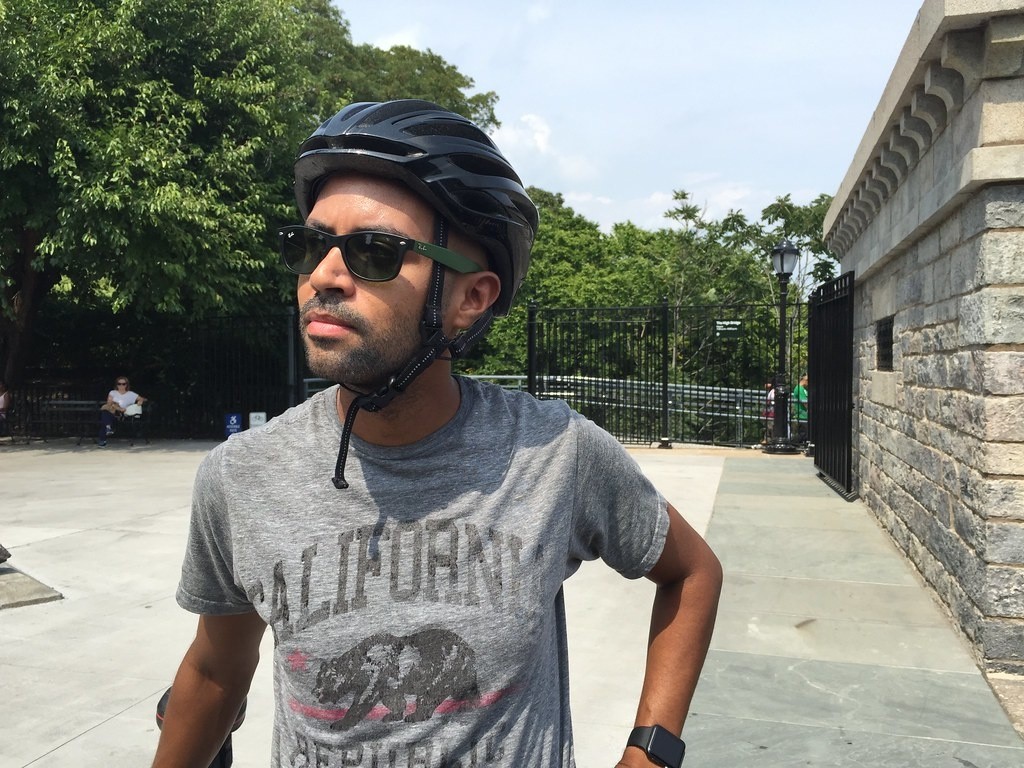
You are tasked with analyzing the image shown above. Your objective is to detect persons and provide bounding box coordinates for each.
[793,372,809,440]
[99,377,144,448]
[153,101,723,768]
[766,385,791,439]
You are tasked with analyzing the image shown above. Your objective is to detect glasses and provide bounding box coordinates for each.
[276,224,485,282]
[117,383,126,385]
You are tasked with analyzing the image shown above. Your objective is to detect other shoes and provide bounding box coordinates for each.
[98,440,107,448]
[105,429,115,436]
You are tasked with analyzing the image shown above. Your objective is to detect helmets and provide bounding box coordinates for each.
[295,99,539,318]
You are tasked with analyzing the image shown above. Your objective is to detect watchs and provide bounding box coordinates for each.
[627,724,686,768]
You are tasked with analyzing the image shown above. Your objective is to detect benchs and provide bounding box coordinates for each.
[24,397,150,447]
[0,392,16,442]
